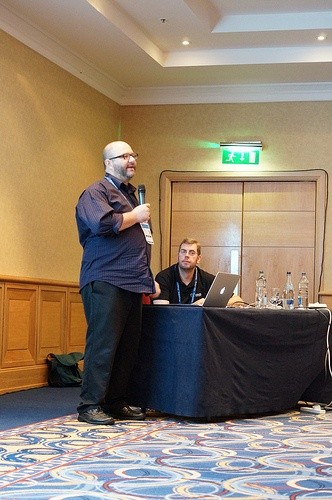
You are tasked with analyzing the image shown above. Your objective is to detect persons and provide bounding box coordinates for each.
[151,239,244,306]
[75,140,161,425]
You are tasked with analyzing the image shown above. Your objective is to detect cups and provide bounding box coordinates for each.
[267,288,281,308]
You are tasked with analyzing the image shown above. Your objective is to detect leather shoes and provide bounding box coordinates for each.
[78,407,114,425]
[117,405,147,419]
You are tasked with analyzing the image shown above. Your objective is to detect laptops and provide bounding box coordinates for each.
[171,272,241,307]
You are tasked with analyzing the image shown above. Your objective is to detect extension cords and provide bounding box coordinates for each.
[300,406,326,414]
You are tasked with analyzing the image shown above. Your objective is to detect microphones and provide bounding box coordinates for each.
[138,184,145,204]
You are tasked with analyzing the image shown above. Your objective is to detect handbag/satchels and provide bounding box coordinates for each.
[47,352,84,386]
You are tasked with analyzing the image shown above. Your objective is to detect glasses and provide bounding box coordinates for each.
[109,153,138,160]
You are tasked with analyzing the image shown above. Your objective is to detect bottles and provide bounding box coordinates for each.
[297,273,309,310]
[283,272,294,309]
[255,271,267,308]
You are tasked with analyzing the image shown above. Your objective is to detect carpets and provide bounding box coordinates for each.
[0,413,332,500]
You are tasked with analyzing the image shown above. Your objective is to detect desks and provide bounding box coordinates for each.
[126,306,332,423]
